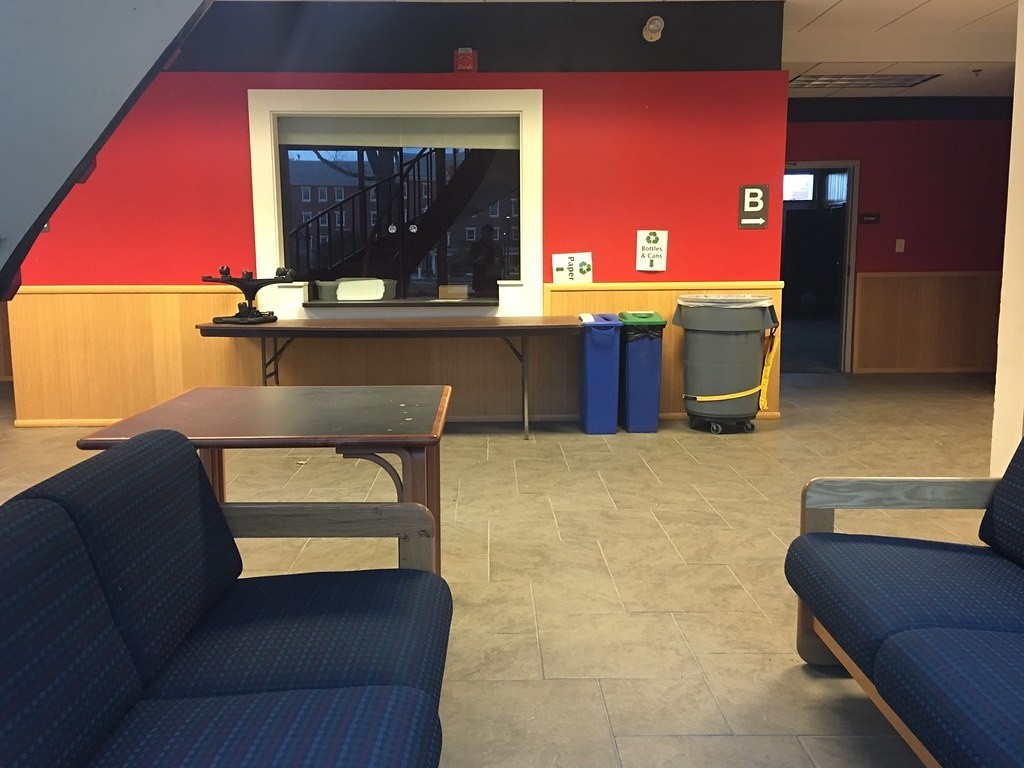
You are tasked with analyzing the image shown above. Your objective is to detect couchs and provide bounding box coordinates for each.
[784,438,1024,768]
[0,428,453,768]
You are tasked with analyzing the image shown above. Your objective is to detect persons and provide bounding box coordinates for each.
[468,224,504,293]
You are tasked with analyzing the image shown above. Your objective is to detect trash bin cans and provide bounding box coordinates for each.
[677,293,780,435]
[617,311,667,433]
[578,312,623,434]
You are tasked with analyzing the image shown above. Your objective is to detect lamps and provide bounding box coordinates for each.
[643,15,665,43]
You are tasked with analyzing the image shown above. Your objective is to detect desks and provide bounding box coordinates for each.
[195,316,582,440]
[77,384,452,584]
